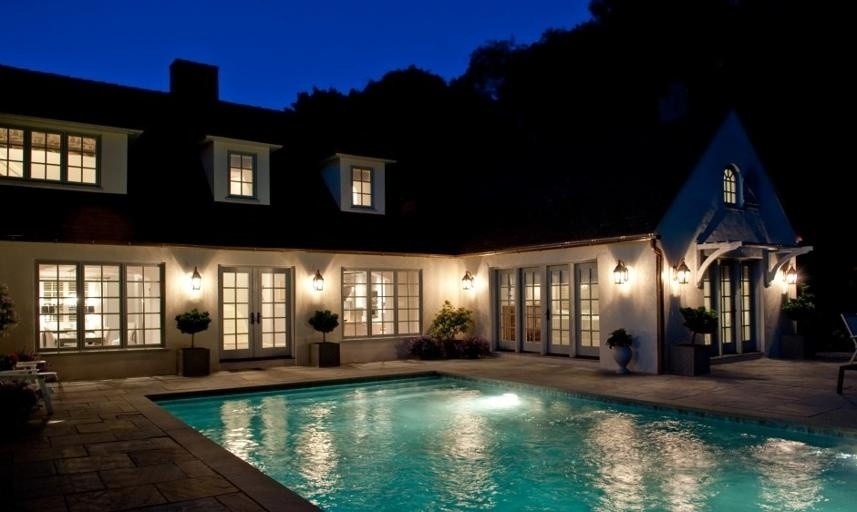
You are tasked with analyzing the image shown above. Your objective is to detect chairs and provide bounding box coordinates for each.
[836,313,856,393]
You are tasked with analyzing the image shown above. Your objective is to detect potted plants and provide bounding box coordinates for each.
[678,303,720,374]
[607,329,638,372]
[310,308,343,368]
[173,307,212,380]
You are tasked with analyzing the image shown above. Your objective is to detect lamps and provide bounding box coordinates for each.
[460,270,477,294]
[189,264,205,294]
[612,259,629,288]
[312,268,328,294]
[676,262,691,286]
[785,263,801,287]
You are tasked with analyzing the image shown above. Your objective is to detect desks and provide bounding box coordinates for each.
[2,357,64,427]
